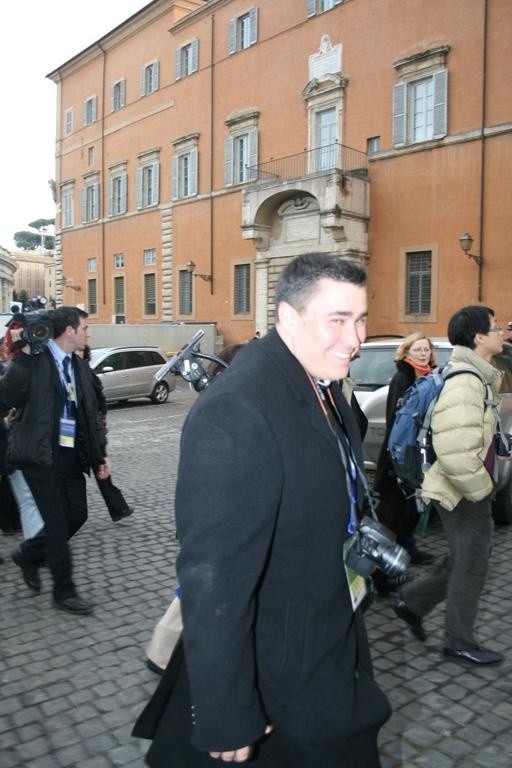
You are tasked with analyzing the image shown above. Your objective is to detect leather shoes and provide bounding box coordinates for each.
[11,545,44,592]
[393,599,427,643]
[439,643,503,666]
[51,590,97,617]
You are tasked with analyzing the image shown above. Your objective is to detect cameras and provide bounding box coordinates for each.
[344,516,414,583]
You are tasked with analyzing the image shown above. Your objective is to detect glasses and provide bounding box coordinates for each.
[490,325,501,333]
[410,348,431,354]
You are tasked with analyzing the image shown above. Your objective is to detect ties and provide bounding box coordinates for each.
[62,356,77,420]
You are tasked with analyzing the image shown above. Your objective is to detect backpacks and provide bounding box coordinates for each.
[386,360,487,499]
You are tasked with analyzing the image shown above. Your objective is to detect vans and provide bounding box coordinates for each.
[89,345,176,404]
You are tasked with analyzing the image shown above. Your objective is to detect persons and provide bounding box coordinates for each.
[374,332,437,562]
[0,303,112,613]
[136,349,414,766]
[130,253,392,768]
[385,306,505,666]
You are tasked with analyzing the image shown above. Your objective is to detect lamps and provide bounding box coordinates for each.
[457,232,482,268]
[186,260,213,282]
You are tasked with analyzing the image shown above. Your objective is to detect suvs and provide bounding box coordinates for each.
[347,336,511,524]
